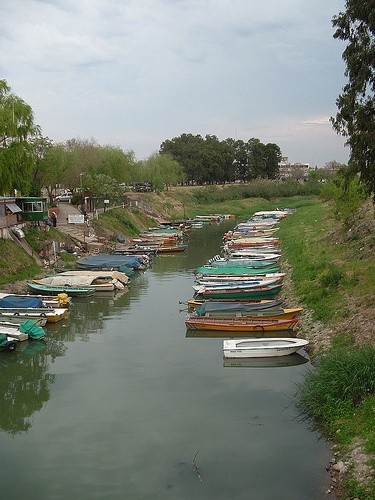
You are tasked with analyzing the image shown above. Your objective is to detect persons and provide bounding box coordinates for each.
[51,213,56,227]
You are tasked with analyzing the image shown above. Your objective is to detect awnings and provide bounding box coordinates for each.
[6,204,22,212]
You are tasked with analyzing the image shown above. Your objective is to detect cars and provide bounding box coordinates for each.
[119,182,152,192]
[55,192,73,201]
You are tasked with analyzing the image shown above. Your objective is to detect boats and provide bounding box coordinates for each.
[178,208,303,331]
[223,338,309,358]
[28,253,151,299]
[0,291,74,349]
[109,214,235,256]
[223,352,309,368]
